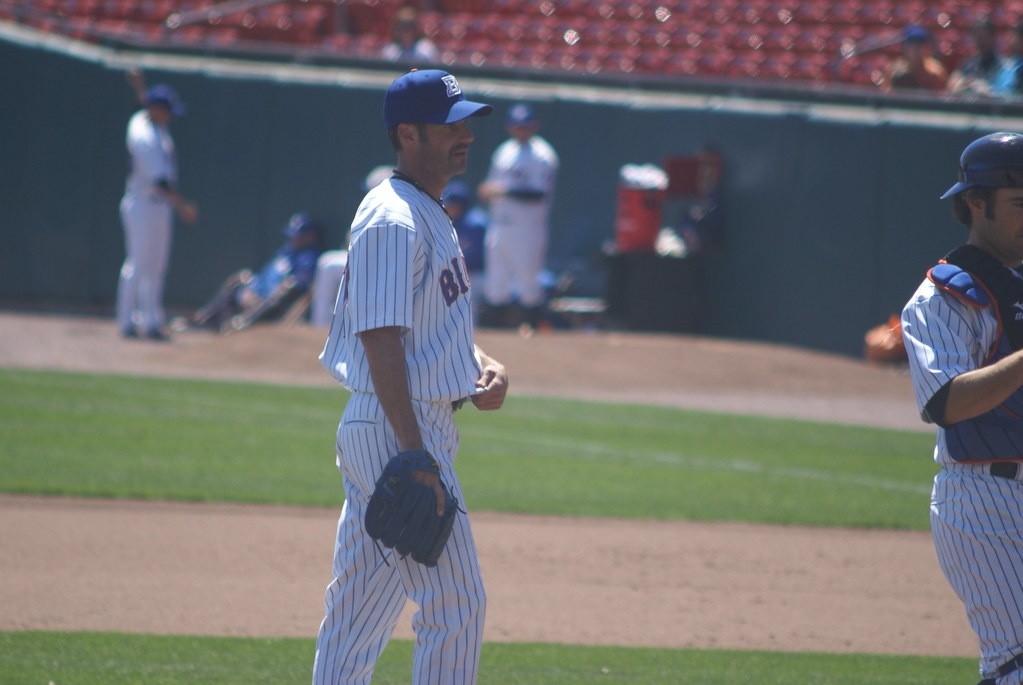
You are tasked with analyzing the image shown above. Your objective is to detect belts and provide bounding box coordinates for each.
[990,462,1018,480]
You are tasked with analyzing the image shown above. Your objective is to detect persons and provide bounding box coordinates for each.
[440,182,490,273]
[116,65,198,342]
[311,68,509,685]
[900,130,1023,685]
[309,247,348,326]
[882,23,948,90]
[173,210,320,334]
[946,17,1023,96]
[476,103,559,314]
[378,6,438,63]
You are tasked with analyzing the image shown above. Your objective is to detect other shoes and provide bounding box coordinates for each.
[120,323,138,337]
[147,328,170,341]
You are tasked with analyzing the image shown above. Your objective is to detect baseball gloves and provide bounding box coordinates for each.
[365,448,469,570]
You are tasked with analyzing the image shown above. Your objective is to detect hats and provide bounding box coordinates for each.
[442,179,469,203]
[506,103,533,124]
[284,212,313,238]
[383,68,494,129]
[147,85,185,116]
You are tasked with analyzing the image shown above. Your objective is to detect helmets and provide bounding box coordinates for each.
[939,133,1023,200]
